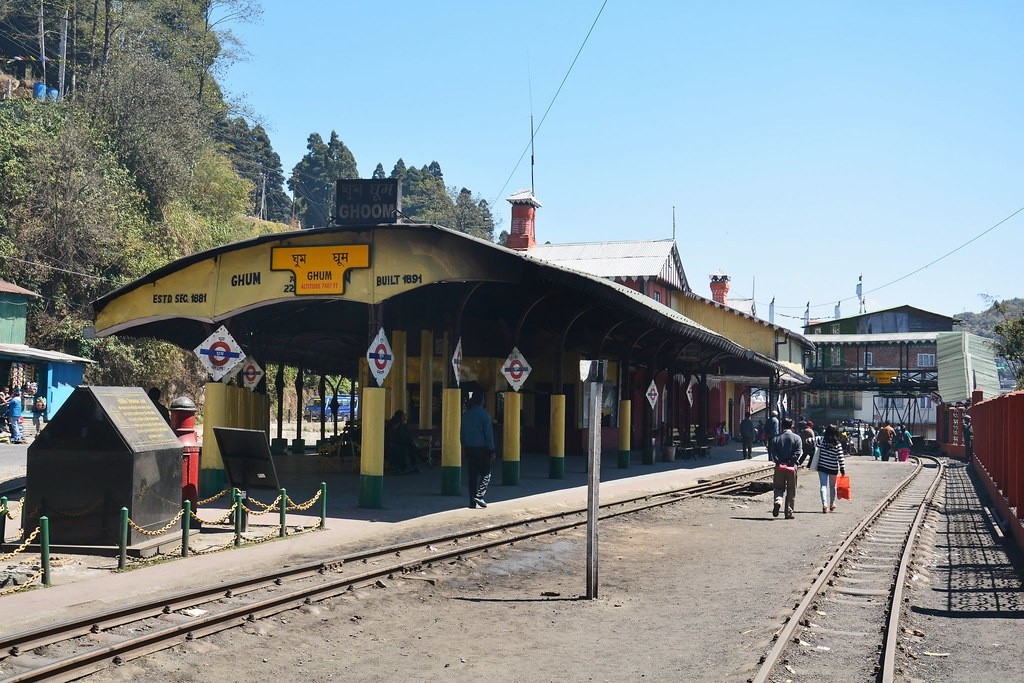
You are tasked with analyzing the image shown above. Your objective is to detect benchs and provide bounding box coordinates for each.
[351,428,433,476]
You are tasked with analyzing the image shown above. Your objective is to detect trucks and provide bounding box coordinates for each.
[303,395,333,422]
[839,425,880,454]
[323,393,359,422]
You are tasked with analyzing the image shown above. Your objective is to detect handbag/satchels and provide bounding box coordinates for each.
[874,447,881,457]
[907,431,913,447]
[810,436,821,472]
[836,474,851,500]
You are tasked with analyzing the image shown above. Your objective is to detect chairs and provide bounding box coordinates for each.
[688,423,713,460]
[671,427,699,460]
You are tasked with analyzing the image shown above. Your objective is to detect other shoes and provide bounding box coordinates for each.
[830,504,836,511]
[823,506,829,513]
[772,503,780,517]
[473,498,487,508]
[785,513,795,519]
[469,505,476,508]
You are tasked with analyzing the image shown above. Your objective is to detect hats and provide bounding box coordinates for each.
[807,421,813,426]
[771,411,778,416]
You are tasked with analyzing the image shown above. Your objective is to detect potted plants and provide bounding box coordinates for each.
[663,425,676,461]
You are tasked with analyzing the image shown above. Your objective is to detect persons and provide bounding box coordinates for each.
[0,386,23,443]
[713,421,731,446]
[771,417,804,519]
[962,414,974,464]
[757,410,913,468]
[805,423,845,513]
[384,410,432,474]
[740,412,755,459]
[459,388,496,509]
[147,387,170,426]
